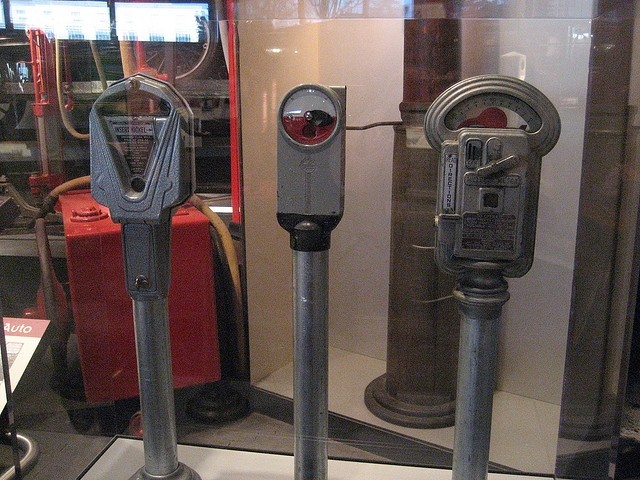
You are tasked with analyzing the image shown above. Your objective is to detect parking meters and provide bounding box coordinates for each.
[277,84,346,480]
[424,74,561,480]
[89,72,202,480]
